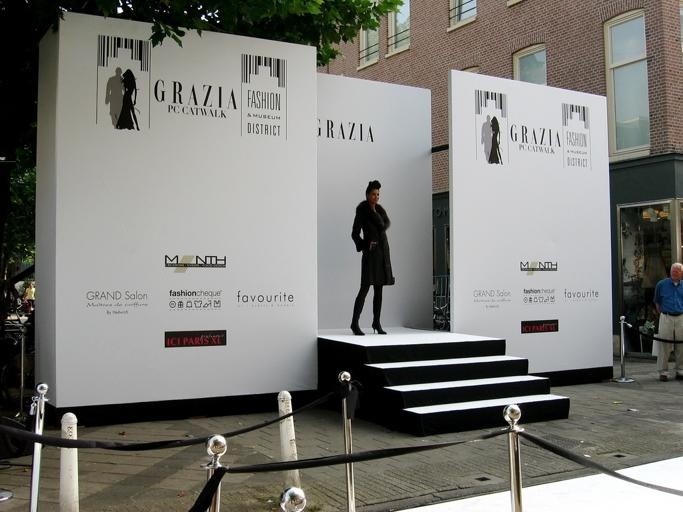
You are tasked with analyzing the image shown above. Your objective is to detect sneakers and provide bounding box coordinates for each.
[675,375,683,380]
[660,375,669,382]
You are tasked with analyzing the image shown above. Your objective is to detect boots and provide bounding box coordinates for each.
[350,305,365,335]
[372,304,386,334]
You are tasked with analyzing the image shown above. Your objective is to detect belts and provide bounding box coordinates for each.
[662,311,681,316]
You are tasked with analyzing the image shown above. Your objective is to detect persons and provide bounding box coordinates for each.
[653,262,682,381]
[23,281,35,310]
[349,179,395,336]
